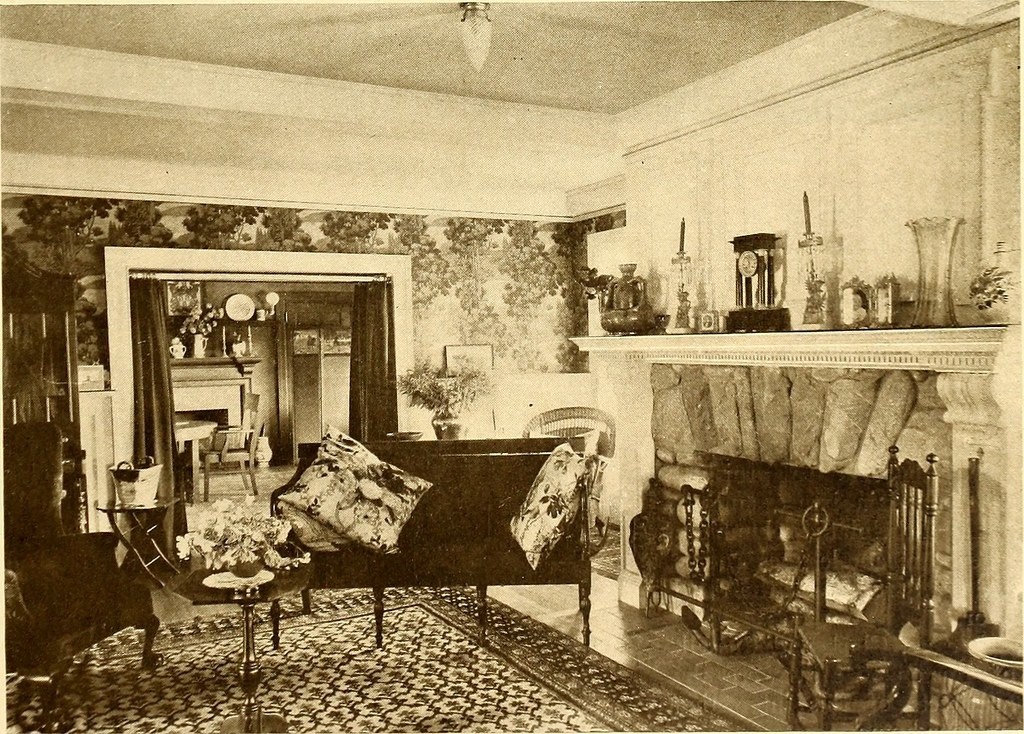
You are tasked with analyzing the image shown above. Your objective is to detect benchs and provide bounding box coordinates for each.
[271,437,592,650]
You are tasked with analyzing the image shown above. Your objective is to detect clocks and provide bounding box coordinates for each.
[727,234,791,332]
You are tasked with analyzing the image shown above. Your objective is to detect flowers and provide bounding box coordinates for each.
[176,493,310,570]
[172,303,224,345]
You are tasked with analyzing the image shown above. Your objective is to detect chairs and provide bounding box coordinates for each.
[787,445,940,732]
[3,421,164,734]
[522,407,615,557]
[200,393,263,501]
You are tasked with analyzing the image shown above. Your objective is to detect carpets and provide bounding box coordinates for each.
[6,586,759,733]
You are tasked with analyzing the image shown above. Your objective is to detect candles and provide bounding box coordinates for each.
[803,191,811,233]
[248,326,251,339]
[223,326,226,348]
[680,217,685,251]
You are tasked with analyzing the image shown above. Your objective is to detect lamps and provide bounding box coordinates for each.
[460,2,493,74]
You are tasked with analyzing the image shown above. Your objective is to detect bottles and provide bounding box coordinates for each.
[599,264,656,334]
[906,218,964,326]
[255,438,272,467]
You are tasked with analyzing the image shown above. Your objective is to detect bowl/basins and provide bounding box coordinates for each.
[386,432,424,441]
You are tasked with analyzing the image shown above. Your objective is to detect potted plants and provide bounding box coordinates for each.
[390,354,495,440]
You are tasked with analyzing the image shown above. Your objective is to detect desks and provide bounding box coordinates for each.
[96,497,181,589]
[176,420,217,503]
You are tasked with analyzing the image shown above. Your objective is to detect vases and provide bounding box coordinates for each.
[228,559,266,579]
[905,217,967,328]
[194,334,208,358]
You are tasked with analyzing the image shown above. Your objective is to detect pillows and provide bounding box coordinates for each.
[509,442,589,571]
[277,426,433,556]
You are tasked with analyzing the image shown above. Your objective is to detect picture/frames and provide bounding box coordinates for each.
[163,280,207,317]
[445,344,494,378]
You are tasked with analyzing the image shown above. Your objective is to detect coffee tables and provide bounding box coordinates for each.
[174,562,313,734]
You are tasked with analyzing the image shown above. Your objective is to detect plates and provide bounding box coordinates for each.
[202,572,273,590]
